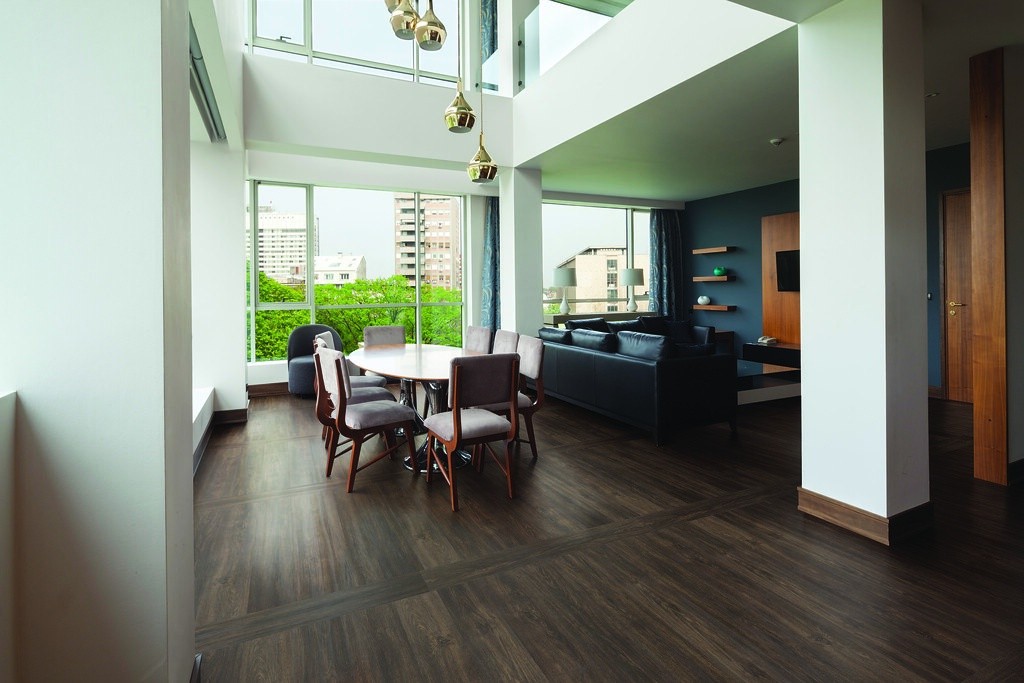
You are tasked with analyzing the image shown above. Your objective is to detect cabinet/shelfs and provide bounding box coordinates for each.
[358,342,400,383]
[692,246,737,312]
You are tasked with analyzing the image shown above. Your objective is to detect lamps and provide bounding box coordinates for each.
[385,0,412,14]
[466,0,498,183]
[390,0,420,40]
[620,269,644,311]
[444,0,477,132]
[414,0,447,51]
[552,268,577,315]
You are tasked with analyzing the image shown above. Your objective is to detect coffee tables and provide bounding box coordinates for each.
[736,359,801,405]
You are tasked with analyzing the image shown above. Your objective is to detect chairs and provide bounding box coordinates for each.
[492,329,519,354]
[314,331,388,438]
[313,337,399,463]
[423,352,521,512]
[365,326,417,413]
[313,346,420,493]
[471,334,546,466]
[421,326,493,421]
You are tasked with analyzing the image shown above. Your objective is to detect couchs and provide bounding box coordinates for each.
[565,316,716,356]
[526,328,738,447]
[287,324,344,398]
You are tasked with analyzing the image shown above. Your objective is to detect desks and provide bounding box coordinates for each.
[348,343,484,472]
[743,343,800,367]
[715,330,735,357]
[554,312,657,328]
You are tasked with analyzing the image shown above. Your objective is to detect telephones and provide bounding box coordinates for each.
[757,335,777,344]
